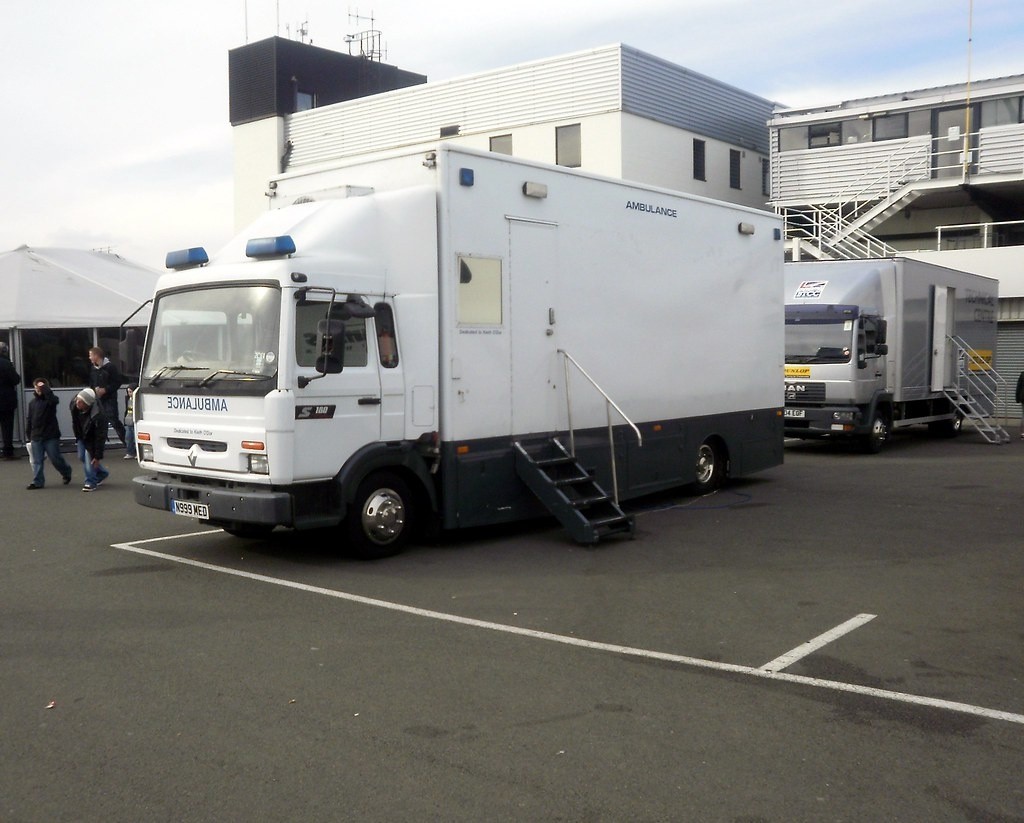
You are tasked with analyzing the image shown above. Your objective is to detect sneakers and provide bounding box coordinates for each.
[97,473,111,485]
[82,484,97,491]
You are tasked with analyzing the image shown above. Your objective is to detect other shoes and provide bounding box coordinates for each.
[135,456,137,459]
[0,450,14,457]
[63,477,71,484]
[26,484,44,489]
[124,454,134,458]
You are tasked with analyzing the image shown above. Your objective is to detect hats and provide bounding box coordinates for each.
[76,388,95,406]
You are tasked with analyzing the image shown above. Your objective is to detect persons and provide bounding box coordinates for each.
[70,387,110,491]
[0,342,21,459]
[1016,371,1024,440]
[88,347,127,447]
[123,384,138,460]
[26,377,72,489]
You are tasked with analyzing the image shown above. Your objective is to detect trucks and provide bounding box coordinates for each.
[784,257,999,454]
[117,141,785,562]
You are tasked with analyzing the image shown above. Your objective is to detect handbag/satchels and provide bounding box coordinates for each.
[26,443,49,472]
